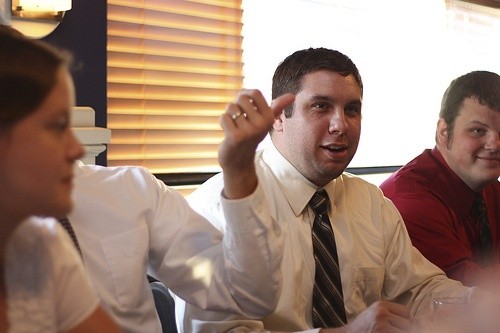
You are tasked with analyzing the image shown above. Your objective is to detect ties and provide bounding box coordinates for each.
[57,214,84,264]
[475,195,492,264]
[308,190,347,330]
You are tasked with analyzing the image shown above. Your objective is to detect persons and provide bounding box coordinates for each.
[169,46,500,333]
[0,23,122,333]
[0,88,295,333]
[379,71,500,292]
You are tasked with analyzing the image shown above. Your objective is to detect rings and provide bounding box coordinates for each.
[232,111,242,120]
